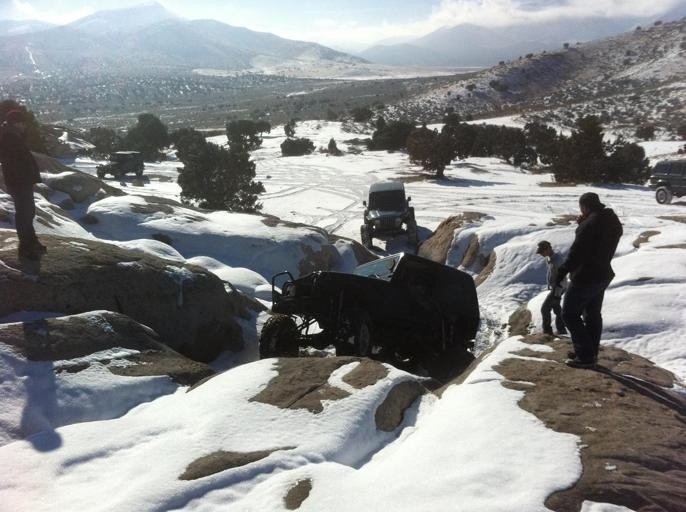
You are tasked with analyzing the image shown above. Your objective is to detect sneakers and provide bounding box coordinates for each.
[554,328,568,336]
[566,353,597,368]
[568,350,599,362]
[31,239,47,252]
[18,247,40,260]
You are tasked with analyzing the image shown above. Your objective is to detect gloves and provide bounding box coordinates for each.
[552,266,568,286]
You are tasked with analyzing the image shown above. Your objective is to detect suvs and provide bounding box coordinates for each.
[649,159,685,203]
[259,252,480,375]
[360,182,416,249]
[96,151,144,180]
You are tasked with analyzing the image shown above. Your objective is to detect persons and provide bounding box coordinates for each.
[535,240,569,335]
[0,109,48,260]
[549,191,624,367]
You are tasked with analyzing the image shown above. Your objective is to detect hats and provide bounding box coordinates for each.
[535,241,550,253]
[579,193,604,213]
[6,110,26,123]
